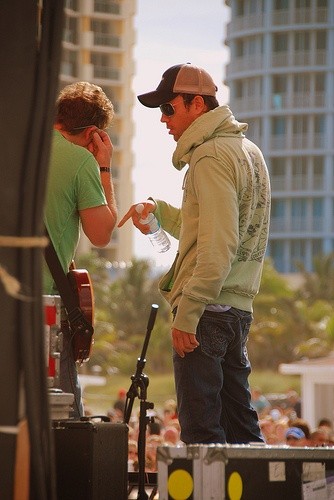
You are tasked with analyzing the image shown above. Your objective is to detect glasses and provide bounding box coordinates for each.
[159,99,189,118]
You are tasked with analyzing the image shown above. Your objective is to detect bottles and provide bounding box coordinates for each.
[135,204,171,253]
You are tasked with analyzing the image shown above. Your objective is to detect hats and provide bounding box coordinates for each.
[137,62,218,108]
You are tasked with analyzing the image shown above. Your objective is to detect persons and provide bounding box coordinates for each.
[82,388,334,473]
[118,63,270,500]
[42,81,118,418]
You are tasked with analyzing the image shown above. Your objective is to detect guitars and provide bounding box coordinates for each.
[65,259,96,365]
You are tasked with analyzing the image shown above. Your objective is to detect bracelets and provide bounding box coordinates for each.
[100,167,111,172]
